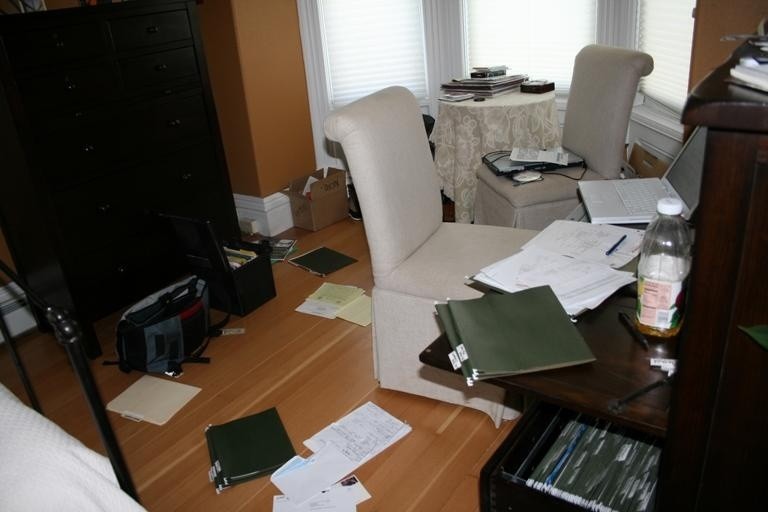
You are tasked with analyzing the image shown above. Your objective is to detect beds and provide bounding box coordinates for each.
[0,259,157,512]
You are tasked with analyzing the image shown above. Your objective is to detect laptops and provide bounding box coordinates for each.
[481,145,584,177]
[577,122,708,225]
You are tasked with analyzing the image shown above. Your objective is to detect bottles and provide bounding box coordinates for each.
[633,194,693,337]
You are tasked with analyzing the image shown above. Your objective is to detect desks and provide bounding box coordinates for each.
[419,37,765,511]
[428,92,562,224]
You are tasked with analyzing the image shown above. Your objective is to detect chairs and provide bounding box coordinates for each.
[472,44,654,228]
[322,85,545,430]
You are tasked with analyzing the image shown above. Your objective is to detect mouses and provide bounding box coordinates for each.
[513,170,543,183]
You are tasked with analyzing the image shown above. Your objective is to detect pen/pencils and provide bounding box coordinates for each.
[619,377,672,405]
[606,235,627,255]
[618,311,649,349]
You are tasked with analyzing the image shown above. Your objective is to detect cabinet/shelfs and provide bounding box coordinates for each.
[0,1,278,334]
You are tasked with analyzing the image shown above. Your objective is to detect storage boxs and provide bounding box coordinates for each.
[279,167,346,233]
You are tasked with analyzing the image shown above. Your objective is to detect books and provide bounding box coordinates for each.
[442,76,526,97]
